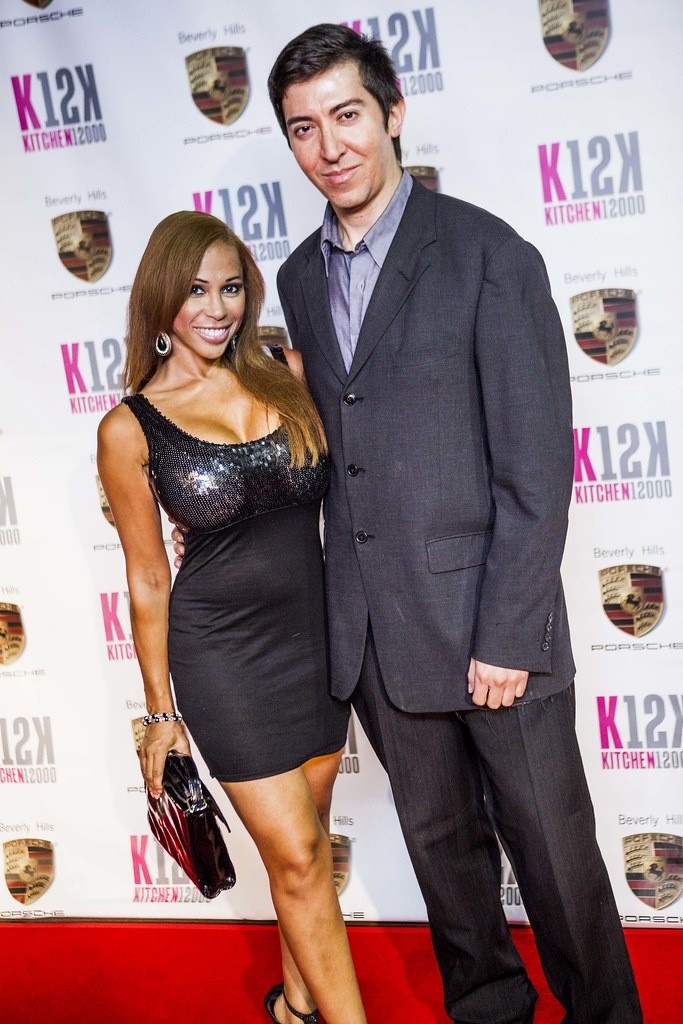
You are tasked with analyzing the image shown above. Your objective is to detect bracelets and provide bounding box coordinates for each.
[142,712,182,727]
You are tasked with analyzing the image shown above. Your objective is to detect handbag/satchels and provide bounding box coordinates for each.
[144,752,237,899]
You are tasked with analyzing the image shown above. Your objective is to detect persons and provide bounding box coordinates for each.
[173,22,645,1024]
[97,212,367,1024]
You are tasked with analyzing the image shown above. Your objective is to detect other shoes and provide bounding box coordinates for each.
[265,982,319,1024]
